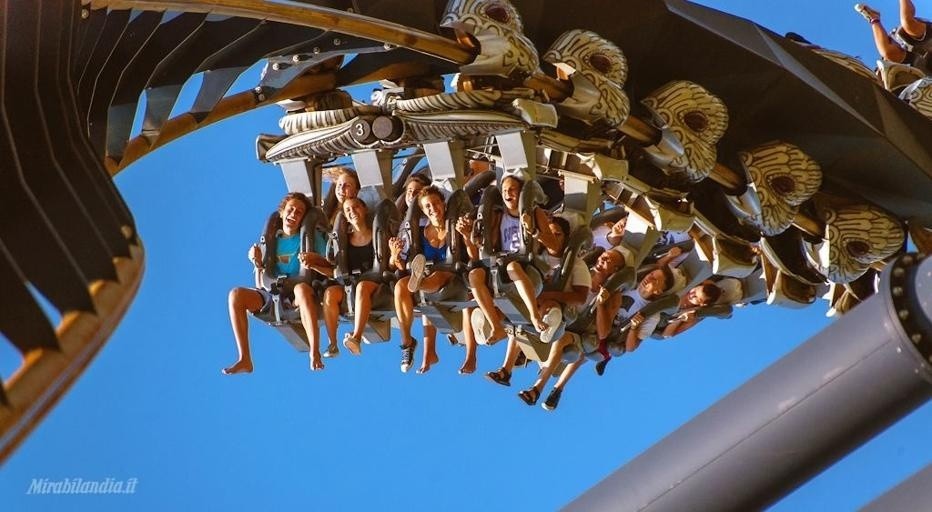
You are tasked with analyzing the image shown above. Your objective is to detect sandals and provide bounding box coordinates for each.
[485,368,512,387]
[518,387,540,406]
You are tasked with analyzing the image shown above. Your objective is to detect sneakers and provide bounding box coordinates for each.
[470,308,491,345]
[539,308,565,345]
[399,337,417,373]
[342,333,362,355]
[597,353,613,378]
[406,253,426,293]
[541,384,562,410]
[322,344,339,357]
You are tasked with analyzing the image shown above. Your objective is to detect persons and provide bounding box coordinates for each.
[853,0,931,81]
[218,159,722,411]
